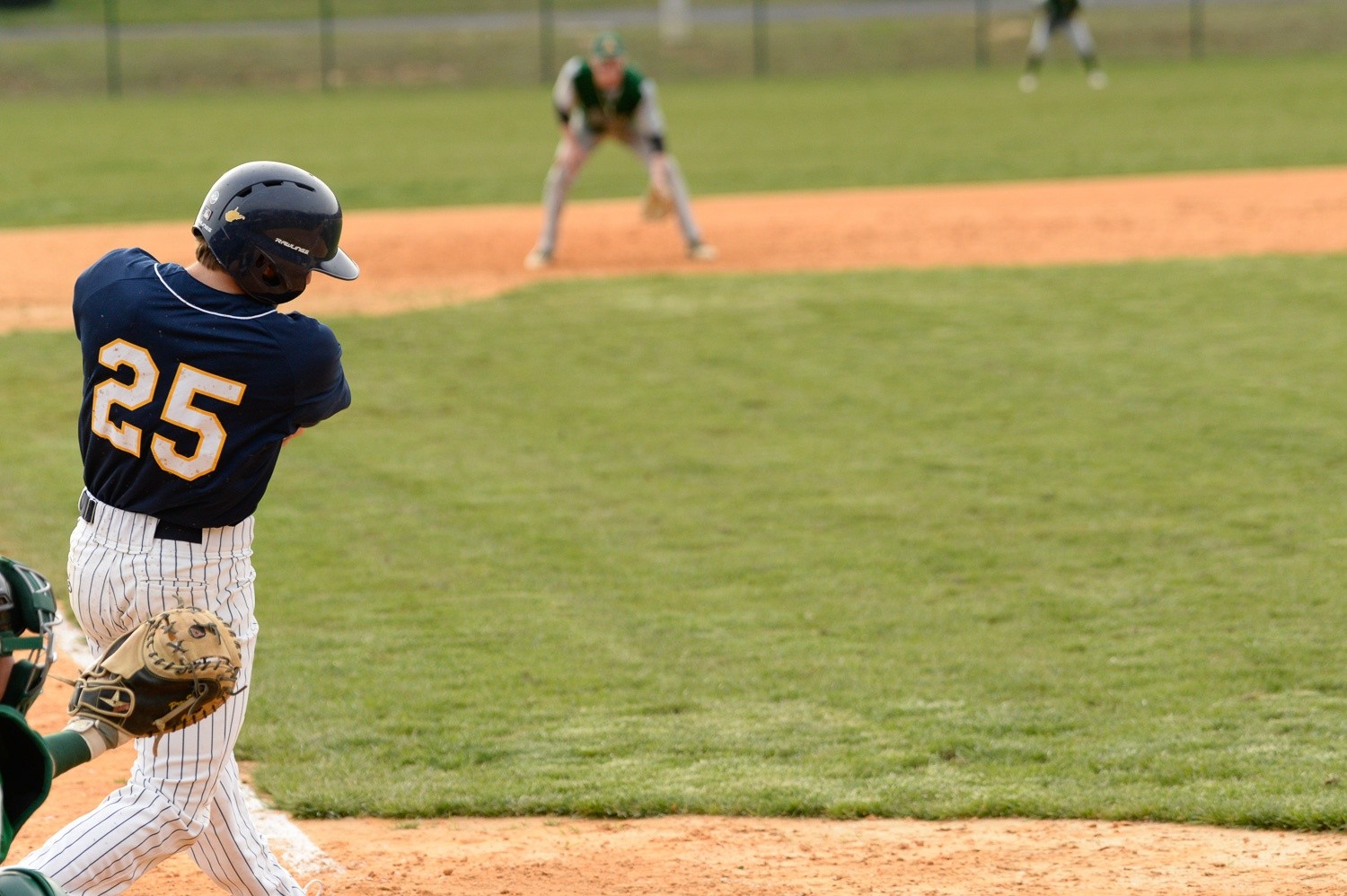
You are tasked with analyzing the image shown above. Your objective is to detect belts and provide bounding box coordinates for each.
[80,493,203,544]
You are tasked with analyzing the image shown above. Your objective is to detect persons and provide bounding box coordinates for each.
[1018,0,1108,94]
[0,160,360,896]
[0,558,242,869]
[526,30,715,272]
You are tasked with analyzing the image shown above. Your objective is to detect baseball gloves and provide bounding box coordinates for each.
[67,604,243,740]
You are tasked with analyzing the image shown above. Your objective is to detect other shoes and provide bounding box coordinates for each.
[686,240,718,263]
[524,246,553,269]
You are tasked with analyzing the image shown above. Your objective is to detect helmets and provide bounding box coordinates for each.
[0,555,61,712]
[592,32,621,62]
[192,160,360,304]
